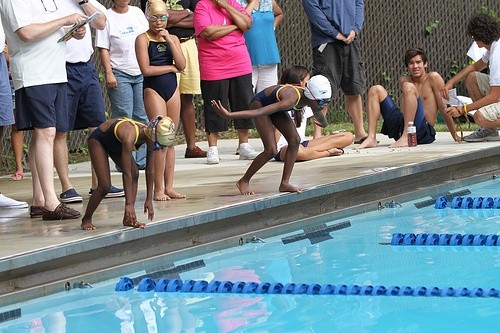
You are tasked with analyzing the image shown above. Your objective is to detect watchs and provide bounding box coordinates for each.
[273,26,276,30]
[79,0,88,6]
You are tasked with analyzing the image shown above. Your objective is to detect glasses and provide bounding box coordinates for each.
[149,15,168,22]
[154,119,168,149]
[41,0,58,13]
[306,86,330,105]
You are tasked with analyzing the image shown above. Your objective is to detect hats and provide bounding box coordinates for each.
[144,116,175,146]
[145,0,169,21]
[304,75,332,100]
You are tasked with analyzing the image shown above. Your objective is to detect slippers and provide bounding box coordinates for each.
[354,135,380,144]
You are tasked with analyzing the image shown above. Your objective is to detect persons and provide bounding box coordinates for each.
[194,0,259,164]
[211,74,332,195]
[79,116,175,230]
[236,0,283,155]
[163,0,207,157]
[0,0,86,220]
[3,40,24,181]
[353,49,464,150]
[441,14,500,142]
[0,17,29,209]
[93,0,150,172]
[270,65,355,161]
[16,203,467,333]
[135,0,186,201]
[302,0,379,145]
[55,0,125,204]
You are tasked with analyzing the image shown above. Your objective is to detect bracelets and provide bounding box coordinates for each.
[186,8,190,14]
[463,104,467,115]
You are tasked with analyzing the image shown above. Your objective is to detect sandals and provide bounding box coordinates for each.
[327,147,344,156]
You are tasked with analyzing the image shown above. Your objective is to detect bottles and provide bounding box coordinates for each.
[407,121,417,147]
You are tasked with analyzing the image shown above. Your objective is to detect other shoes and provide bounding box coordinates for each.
[239,145,256,160]
[88,184,125,199]
[60,188,82,202]
[0,193,28,208]
[207,146,219,164]
[42,203,83,222]
[30,205,42,218]
[185,146,207,158]
[9,173,23,180]
[464,126,500,141]
